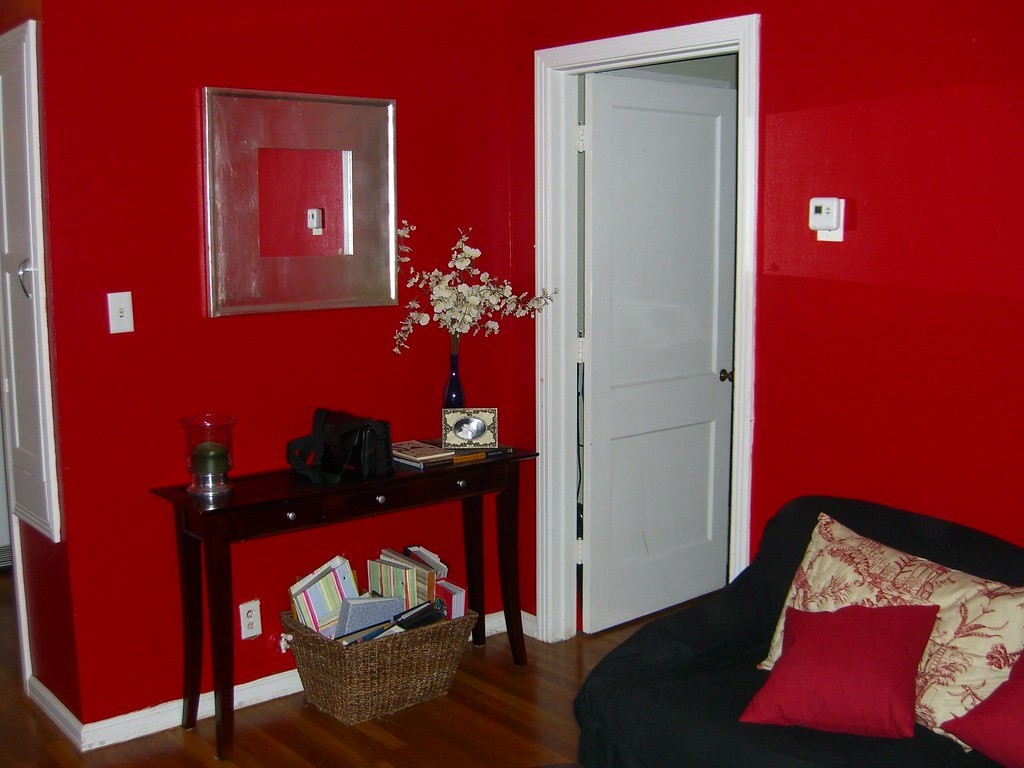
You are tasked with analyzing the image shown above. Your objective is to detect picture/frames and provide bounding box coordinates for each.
[441,409,498,449]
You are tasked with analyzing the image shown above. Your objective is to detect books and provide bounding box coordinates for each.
[391,453,454,469]
[333,620,392,648]
[370,624,406,641]
[393,597,434,623]
[288,555,361,629]
[391,436,457,460]
[293,558,360,640]
[436,577,466,621]
[381,548,436,608]
[358,591,372,598]
[404,611,440,629]
[333,593,406,640]
[403,543,449,580]
[375,553,419,608]
[368,557,417,610]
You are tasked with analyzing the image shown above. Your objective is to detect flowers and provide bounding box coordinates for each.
[387,218,553,356]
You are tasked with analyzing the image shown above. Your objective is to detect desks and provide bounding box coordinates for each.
[148,424,543,762]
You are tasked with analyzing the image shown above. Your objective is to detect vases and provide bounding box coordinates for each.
[441,353,464,409]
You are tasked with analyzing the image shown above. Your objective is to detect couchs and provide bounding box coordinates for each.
[573,490,1024,768]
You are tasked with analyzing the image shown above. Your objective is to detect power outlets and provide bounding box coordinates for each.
[239,599,263,640]
[107,291,134,334]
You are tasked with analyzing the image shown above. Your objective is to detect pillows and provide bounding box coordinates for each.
[736,507,1022,767]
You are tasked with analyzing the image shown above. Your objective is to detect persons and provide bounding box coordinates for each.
[456,424,472,440]
[474,423,486,438]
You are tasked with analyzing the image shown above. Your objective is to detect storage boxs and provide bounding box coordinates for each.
[280,607,478,726]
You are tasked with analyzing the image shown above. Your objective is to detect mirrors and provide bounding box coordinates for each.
[199,88,399,319]
